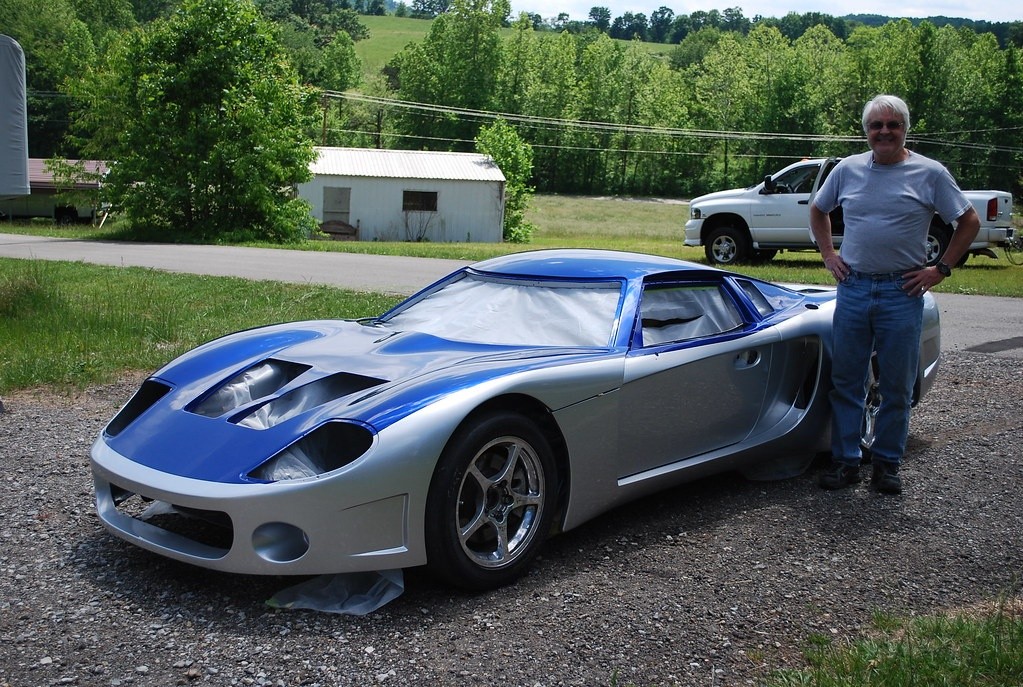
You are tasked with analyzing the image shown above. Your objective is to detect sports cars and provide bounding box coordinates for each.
[90,247,944,591]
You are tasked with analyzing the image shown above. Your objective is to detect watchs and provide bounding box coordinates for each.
[936,262,951,277]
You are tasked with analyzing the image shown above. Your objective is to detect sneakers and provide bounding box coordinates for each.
[870,461,903,496]
[818,456,859,490]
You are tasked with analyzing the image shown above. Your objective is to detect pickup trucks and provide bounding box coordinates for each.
[682,156,1014,268]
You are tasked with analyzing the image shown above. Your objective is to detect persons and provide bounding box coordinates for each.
[810,94,981,495]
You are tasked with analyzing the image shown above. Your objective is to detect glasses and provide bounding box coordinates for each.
[863,120,906,130]
